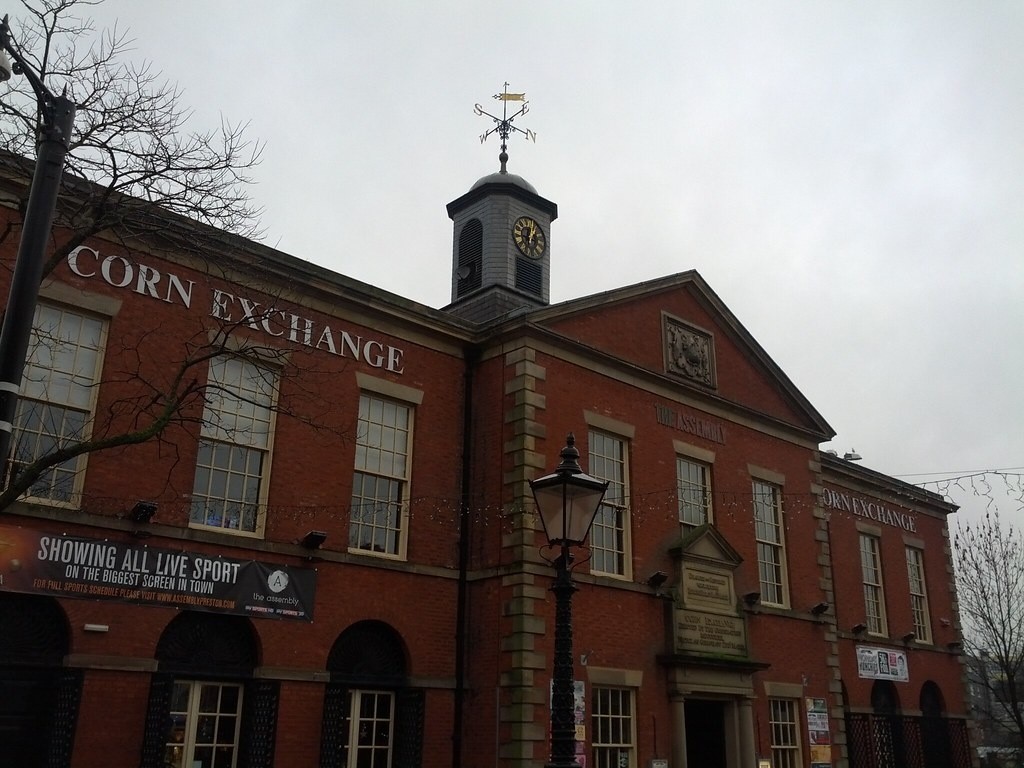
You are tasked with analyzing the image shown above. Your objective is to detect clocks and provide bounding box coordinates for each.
[512,215,547,260]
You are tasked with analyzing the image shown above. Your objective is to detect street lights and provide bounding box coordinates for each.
[526,429,611,768]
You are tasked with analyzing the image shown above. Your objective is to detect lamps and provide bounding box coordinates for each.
[852,622,868,635]
[743,591,761,605]
[299,529,328,550]
[646,571,669,588]
[903,632,915,641]
[580,649,594,666]
[811,602,829,614]
[551,552,575,569]
[802,672,815,686]
[84,624,109,632]
[127,499,159,524]
[948,640,962,648]
